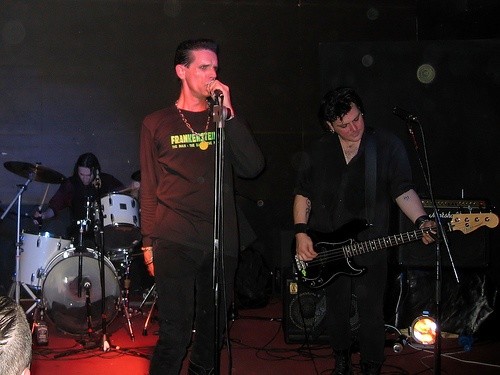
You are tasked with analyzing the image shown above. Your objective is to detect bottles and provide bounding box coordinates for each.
[457,331,473,352]
[393,334,407,353]
[35,306,48,347]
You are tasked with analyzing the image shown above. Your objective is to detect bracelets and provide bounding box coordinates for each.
[414,215,431,228]
[141,247,152,250]
[294,223,308,234]
[145,256,153,266]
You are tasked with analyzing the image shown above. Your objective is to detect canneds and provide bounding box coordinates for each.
[36,325,49,345]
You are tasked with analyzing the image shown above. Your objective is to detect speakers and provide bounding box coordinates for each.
[282,278,361,343]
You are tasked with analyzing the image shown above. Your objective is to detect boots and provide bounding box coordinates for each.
[359,359,386,375]
[330,345,354,375]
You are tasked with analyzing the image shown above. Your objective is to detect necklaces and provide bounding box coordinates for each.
[175,99,211,150]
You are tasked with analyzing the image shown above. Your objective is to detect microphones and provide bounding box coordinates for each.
[392,107,417,121]
[214,89,224,97]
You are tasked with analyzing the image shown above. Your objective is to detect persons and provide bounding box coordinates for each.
[292,85,438,375]
[138,40,265,375]
[34,153,126,249]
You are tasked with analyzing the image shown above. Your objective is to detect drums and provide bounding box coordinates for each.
[91,194,141,250]
[37,247,122,336]
[19,230,75,291]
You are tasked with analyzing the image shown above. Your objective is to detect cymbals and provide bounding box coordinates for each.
[4,161,67,184]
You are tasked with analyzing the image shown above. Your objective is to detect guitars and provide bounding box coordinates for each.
[291,209,499,289]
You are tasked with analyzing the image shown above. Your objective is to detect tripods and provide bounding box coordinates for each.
[52,184,153,362]
